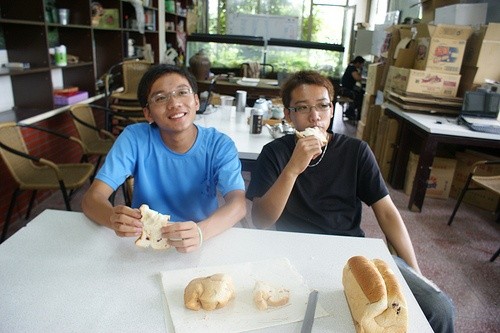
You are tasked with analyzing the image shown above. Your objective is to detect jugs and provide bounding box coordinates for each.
[253,99,273,119]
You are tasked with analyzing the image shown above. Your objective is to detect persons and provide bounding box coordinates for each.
[340,56,367,122]
[244,71,457,333]
[80,64,248,254]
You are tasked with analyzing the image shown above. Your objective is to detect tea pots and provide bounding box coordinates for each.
[265,120,296,140]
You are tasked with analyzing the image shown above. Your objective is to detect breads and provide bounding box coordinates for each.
[184,273,235,310]
[253,278,289,310]
[342,255,408,333]
[135,204,171,250]
[296,126,328,146]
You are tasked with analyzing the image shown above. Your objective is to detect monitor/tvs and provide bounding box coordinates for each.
[462,90,500,119]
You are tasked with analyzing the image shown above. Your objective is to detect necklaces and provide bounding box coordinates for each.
[295,134,330,167]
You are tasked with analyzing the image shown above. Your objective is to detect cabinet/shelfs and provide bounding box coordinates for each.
[0,0,188,125]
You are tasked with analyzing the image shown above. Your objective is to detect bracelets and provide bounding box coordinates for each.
[196,225,205,247]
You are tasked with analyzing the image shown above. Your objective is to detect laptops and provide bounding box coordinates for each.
[196,78,216,114]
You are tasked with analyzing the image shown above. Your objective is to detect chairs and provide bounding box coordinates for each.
[0,60,156,245]
[336,96,354,121]
[447,158,500,263]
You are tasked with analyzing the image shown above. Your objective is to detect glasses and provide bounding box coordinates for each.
[144,86,195,108]
[285,102,331,113]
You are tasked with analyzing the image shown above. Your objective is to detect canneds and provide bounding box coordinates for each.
[250,109,264,135]
[236,91,247,112]
[54,46,68,66]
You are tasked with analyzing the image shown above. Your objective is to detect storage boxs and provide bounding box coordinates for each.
[355,21,500,211]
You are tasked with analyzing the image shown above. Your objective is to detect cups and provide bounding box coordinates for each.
[59,8,69,24]
[271,106,284,119]
[249,109,264,134]
[236,91,247,111]
[221,95,234,121]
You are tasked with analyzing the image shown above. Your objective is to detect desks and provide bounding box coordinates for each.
[384,102,500,213]
[197,75,282,108]
[0,209,435,333]
[193,105,345,172]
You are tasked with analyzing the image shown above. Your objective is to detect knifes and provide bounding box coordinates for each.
[300,291,318,333]
